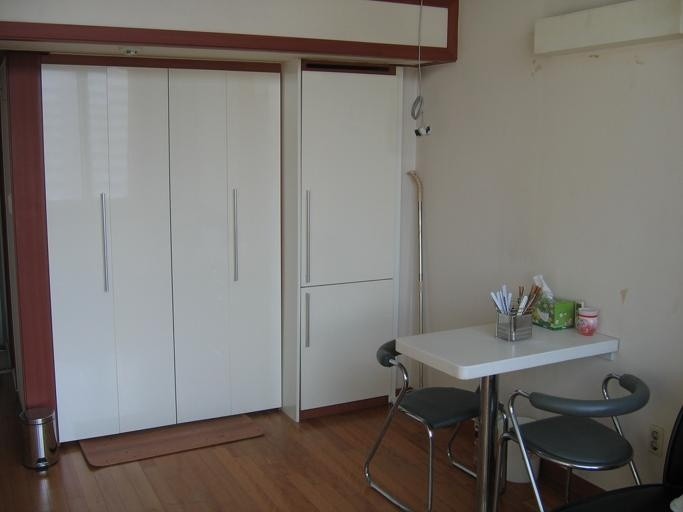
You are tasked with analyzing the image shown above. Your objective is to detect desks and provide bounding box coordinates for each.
[393,317,619,512]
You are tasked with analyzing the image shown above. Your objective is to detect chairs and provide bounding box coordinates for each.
[545,404,683,511]
[485,370,652,512]
[363,337,483,511]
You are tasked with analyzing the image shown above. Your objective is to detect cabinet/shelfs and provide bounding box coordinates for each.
[298,68,401,291]
[171,68,282,430]
[42,63,170,445]
[301,278,397,410]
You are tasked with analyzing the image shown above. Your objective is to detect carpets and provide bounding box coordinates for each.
[77,410,264,471]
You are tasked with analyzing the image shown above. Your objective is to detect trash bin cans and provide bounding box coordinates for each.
[497,417,541,483]
[18,407,60,471]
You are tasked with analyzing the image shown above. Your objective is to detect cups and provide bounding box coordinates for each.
[575,307,601,336]
